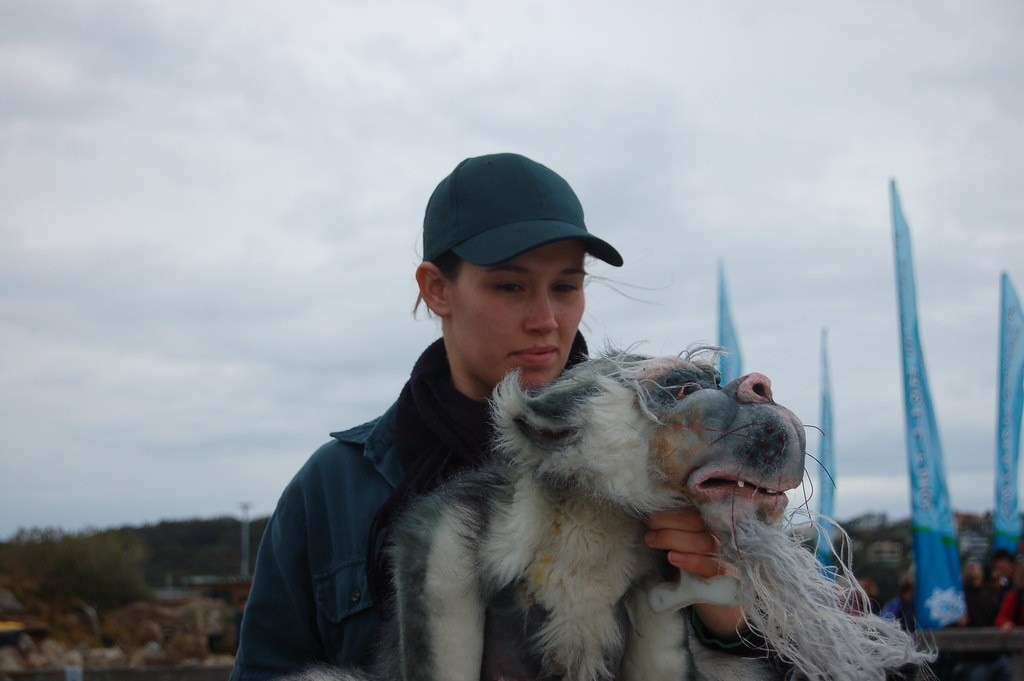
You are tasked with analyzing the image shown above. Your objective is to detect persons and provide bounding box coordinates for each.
[839,548,1024,681]
[230,153,796,681]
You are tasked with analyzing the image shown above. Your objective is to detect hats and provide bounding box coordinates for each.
[424,153,624,273]
